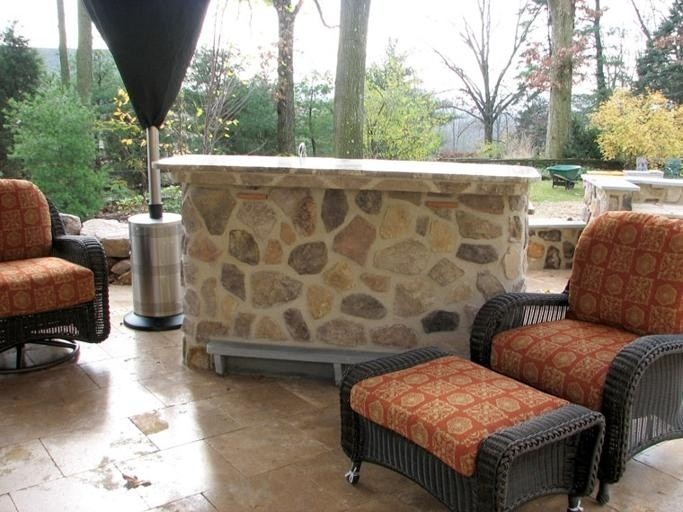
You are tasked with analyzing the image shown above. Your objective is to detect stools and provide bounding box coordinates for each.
[339,346,606,512]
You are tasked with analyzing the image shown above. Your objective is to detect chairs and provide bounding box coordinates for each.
[0,179,111,375]
[470,208,683,507]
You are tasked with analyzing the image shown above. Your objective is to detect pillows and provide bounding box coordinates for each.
[0,177,53,262]
[567,210,683,335]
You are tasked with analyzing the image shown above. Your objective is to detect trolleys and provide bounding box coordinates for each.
[547,165,582,190]
